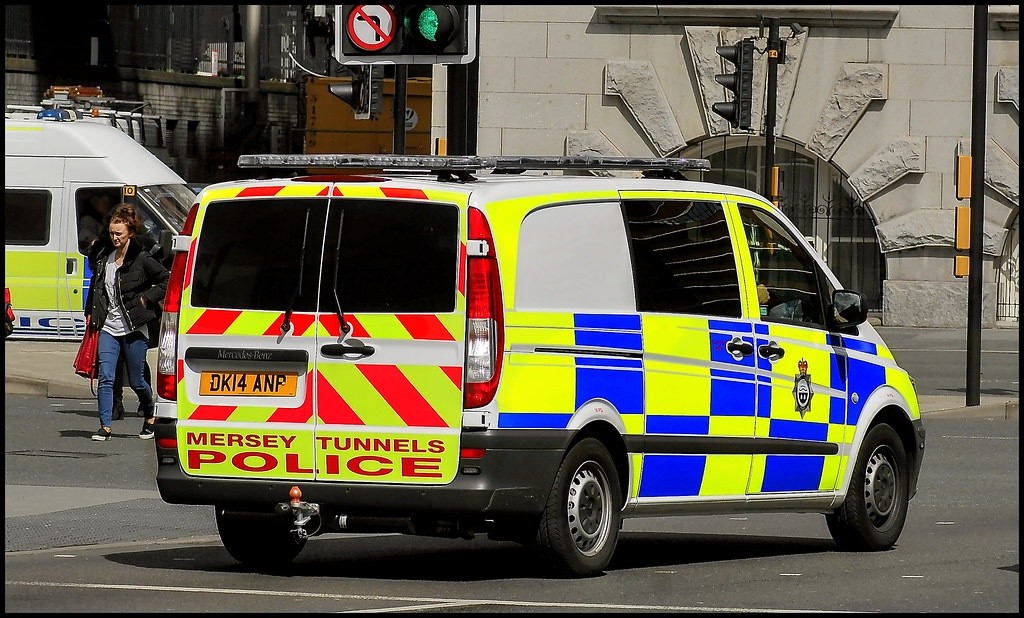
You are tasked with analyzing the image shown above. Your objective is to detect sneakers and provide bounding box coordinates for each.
[139,420,155,439]
[92,428,111,440]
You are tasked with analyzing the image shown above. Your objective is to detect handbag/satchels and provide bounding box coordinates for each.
[73,324,99,396]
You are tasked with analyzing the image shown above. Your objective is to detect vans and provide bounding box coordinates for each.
[152,152,928,580]
[5,83,205,341]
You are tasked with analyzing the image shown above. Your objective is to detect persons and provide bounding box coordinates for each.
[84,203,170,441]
[757,283,770,304]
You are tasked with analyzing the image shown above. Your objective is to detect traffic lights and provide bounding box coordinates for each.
[326,4,384,119]
[402,1,470,57]
[712,39,755,130]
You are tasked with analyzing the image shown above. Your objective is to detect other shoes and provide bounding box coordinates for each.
[138,409,145,417]
[113,407,124,420]
